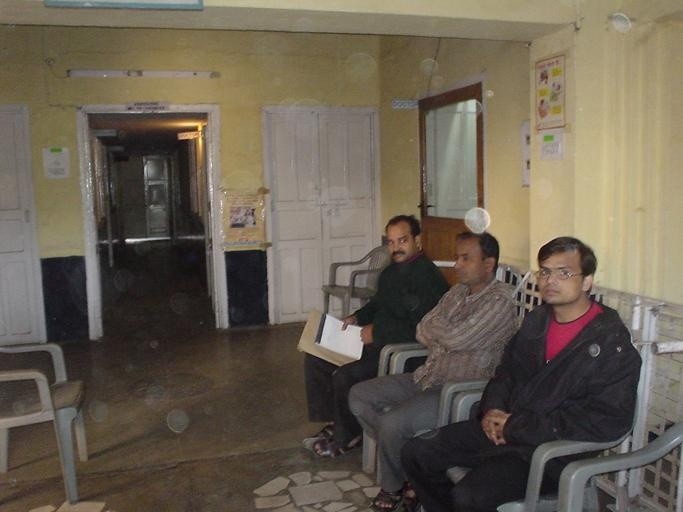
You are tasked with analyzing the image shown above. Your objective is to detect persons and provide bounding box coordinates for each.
[303,215,450,459]
[402,236,643,512]
[348,231,519,512]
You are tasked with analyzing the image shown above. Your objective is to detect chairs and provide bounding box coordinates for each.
[321,244,392,318]
[555,343,683,512]
[391,272,541,429]
[450,284,655,512]
[362,265,520,480]
[0,343,88,505]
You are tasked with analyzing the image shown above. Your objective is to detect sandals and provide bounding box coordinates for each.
[299,423,337,452]
[402,479,423,512]
[312,434,363,459]
[371,487,404,512]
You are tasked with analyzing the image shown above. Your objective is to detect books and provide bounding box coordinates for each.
[298,308,366,367]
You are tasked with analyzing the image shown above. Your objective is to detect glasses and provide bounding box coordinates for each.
[532,269,585,282]
